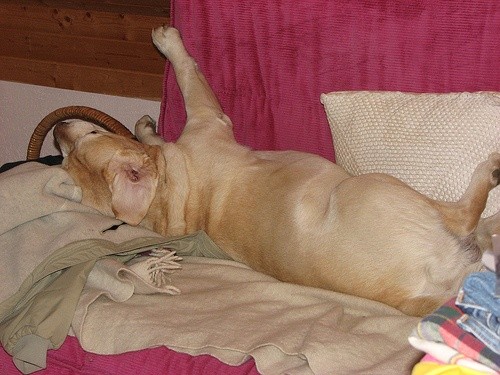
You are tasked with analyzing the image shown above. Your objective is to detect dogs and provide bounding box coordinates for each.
[52,22,500,318]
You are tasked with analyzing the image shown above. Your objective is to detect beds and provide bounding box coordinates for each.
[0,1,500,375]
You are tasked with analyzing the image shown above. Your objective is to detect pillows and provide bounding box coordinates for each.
[318,89,500,225]
